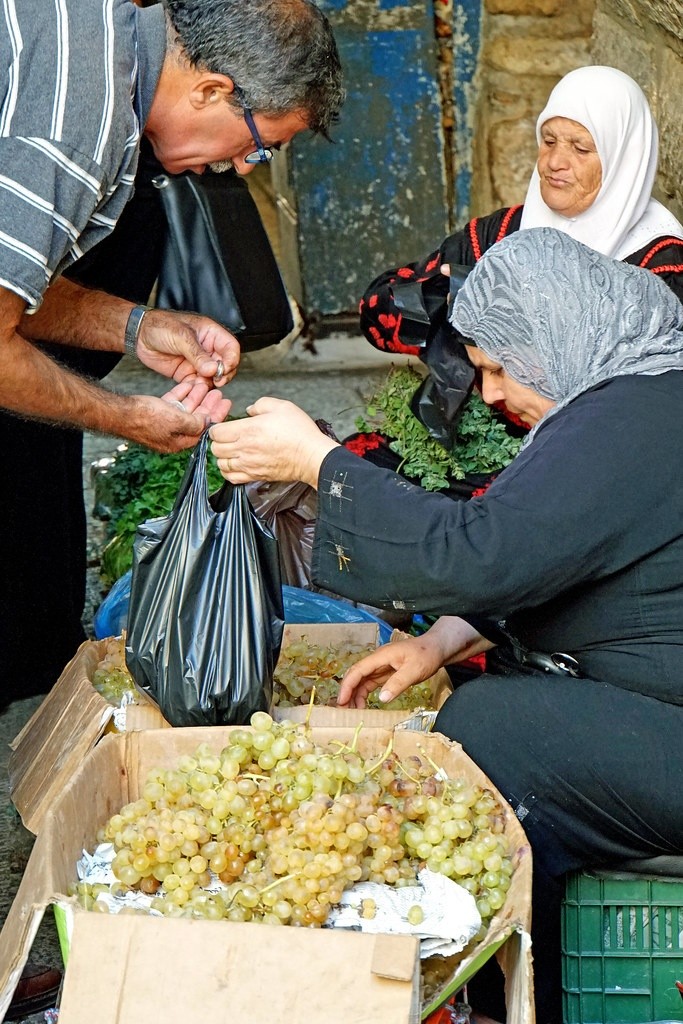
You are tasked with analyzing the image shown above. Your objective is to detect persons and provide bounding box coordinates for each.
[209,224,683,1012]
[0,3,343,713]
[334,63,683,680]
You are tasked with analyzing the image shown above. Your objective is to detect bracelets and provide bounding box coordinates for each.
[123,304,154,359]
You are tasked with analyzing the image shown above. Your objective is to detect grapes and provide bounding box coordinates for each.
[89,634,432,717]
[70,683,512,1014]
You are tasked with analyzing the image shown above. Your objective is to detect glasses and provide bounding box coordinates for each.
[229,80,272,165]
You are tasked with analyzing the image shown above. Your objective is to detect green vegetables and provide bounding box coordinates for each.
[355,359,526,495]
[93,431,228,609]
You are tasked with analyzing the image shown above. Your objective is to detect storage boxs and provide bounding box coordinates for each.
[559,870,683,1024]
[0,621,537,1024]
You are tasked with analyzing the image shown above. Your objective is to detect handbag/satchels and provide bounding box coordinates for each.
[151,170,294,354]
[124,421,281,729]
